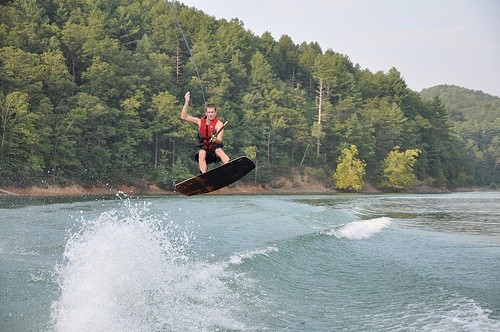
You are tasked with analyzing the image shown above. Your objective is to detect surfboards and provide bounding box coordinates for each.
[175,156,257,197]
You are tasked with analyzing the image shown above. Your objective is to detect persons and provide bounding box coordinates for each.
[180,90,231,175]
